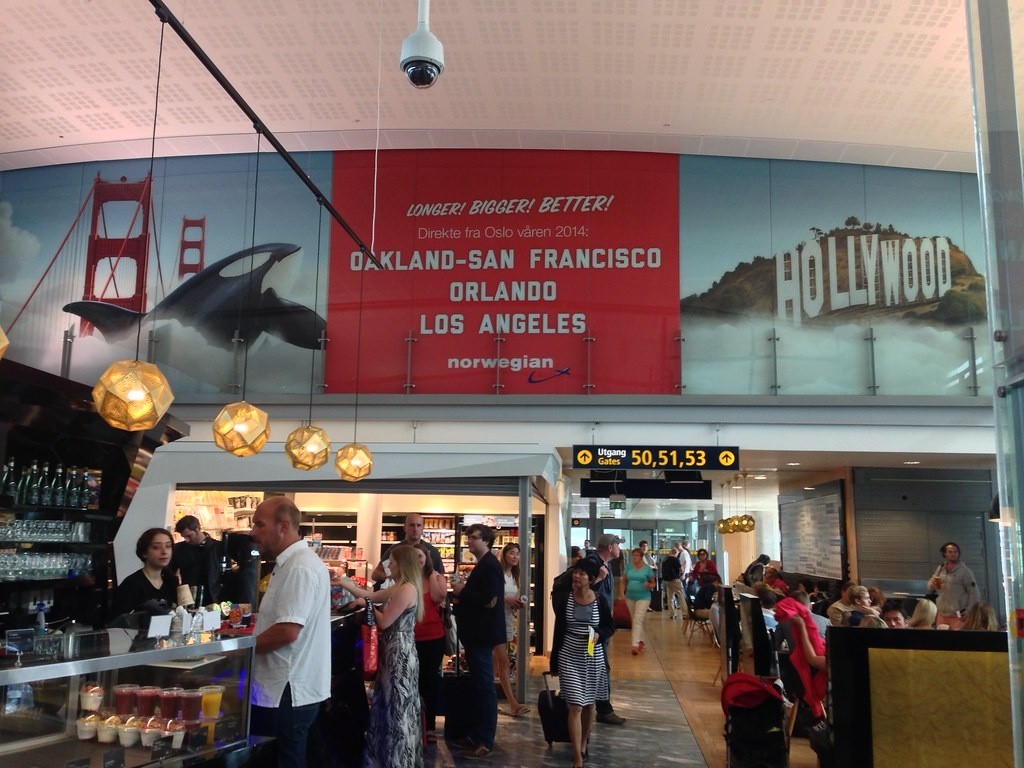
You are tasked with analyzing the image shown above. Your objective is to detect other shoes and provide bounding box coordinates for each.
[580,741,588,760]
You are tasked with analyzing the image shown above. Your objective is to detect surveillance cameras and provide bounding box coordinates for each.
[399,32,445,88]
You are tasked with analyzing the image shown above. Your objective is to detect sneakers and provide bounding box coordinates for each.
[638,641,646,652]
[596,714,626,725]
[631,646,639,655]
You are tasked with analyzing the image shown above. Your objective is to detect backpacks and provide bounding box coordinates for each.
[549,556,610,618]
[662,559,675,580]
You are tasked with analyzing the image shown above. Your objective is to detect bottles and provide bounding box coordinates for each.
[0,455,91,510]
[34,602,45,637]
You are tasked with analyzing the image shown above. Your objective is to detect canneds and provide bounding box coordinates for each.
[383,530,398,541]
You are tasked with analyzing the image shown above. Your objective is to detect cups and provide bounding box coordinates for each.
[76,681,226,754]
[934,576,941,589]
[176,583,196,607]
[0,518,91,542]
[519,595,528,603]
[0,552,92,579]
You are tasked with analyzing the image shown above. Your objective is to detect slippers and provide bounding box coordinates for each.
[463,744,494,760]
[510,705,533,716]
[452,736,478,749]
[427,734,437,744]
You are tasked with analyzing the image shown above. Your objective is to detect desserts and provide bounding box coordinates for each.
[75,713,187,749]
[79,681,104,711]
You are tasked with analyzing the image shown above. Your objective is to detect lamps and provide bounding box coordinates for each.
[286,163,333,472]
[717,471,756,534]
[213,120,272,459]
[89,19,176,432]
[337,216,373,482]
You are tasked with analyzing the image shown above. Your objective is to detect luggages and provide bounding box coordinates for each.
[538,671,573,747]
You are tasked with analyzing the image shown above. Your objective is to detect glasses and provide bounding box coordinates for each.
[508,543,520,547]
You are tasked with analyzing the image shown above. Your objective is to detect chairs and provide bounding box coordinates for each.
[678,587,828,736]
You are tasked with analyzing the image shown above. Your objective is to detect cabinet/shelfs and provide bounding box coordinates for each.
[298,511,460,594]
[456,514,545,654]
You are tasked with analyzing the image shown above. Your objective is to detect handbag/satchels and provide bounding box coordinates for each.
[614,599,632,629]
[360,596,379,682]
[434,573,457,656]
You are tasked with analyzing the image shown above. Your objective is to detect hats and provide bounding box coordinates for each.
[596,534,626,549]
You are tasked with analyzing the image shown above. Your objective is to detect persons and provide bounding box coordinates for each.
[688,548,722,649]
[331,546,425,768]
[170,517,222,606]
[371,513,446,582]
[639,540,658,613]
[575,533,626,728]
[742,555,999,768]
[414,544,447,733]
[662,542,694,620]
[220,496,331,768]
[548,558,615,768]
[452,524,508,760]
[618,547,656,655]
[498,543,531,715]
[106,527,179,689]
[571,540,597,565]
[927,541,981,631]
